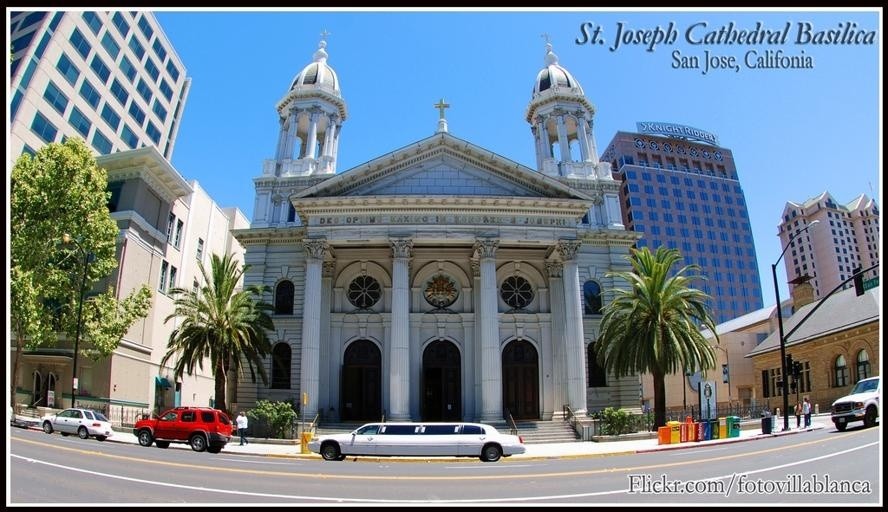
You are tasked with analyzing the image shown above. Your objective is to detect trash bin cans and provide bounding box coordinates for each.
[581,426,590,441]
[760,411,772,434]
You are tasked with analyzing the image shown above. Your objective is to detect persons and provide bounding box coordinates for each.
[236,412,248,445]
[803,398,809,427]
[807,400,811,425]
[794,402,802,427]
